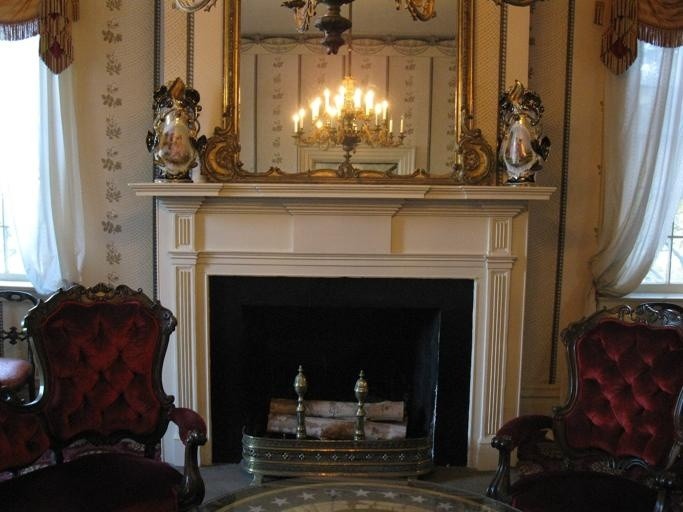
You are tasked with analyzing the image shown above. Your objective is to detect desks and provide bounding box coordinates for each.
[191,476,520,511]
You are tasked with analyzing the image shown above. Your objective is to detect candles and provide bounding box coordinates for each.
[291,85,404,135]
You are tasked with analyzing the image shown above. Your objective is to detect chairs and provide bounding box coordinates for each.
[482,295,683,511]
[1,278,212,512]
